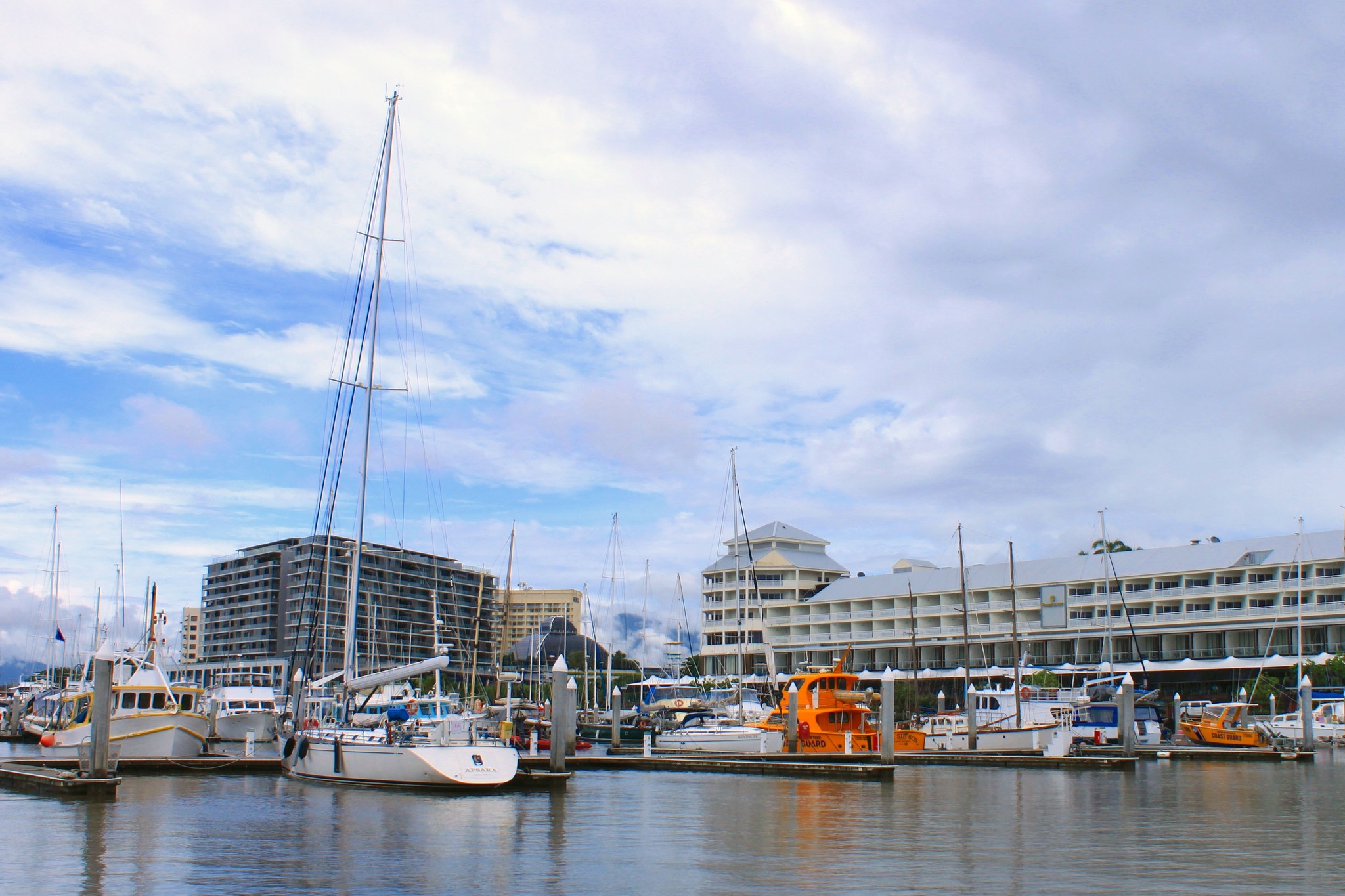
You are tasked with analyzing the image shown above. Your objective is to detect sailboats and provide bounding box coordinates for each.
[424,448,1159,759]
[0,477,101,738]
[282,90,521,791]
[1234,516,1345,740]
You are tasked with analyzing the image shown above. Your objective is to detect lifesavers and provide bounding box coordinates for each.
[303,718,319,730]
[639,721,647,727]
[1020,686,1031,698]
[1049,688,1058,699]
[407,699,418,715]
[675,699,684,707]
[32,710,37,716]
[474,699,482,714]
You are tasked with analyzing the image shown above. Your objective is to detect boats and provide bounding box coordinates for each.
[1298,690,1345,720]
[736,642,927,754]
[1183,702,1269,748]
[206,671,280,744]
[37,581,210,768]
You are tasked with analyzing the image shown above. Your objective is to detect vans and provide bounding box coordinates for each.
[1179,701,1213,719]
